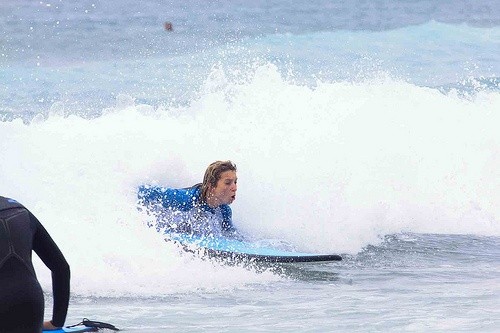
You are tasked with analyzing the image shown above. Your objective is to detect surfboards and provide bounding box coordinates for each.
[157,228,342,263]
